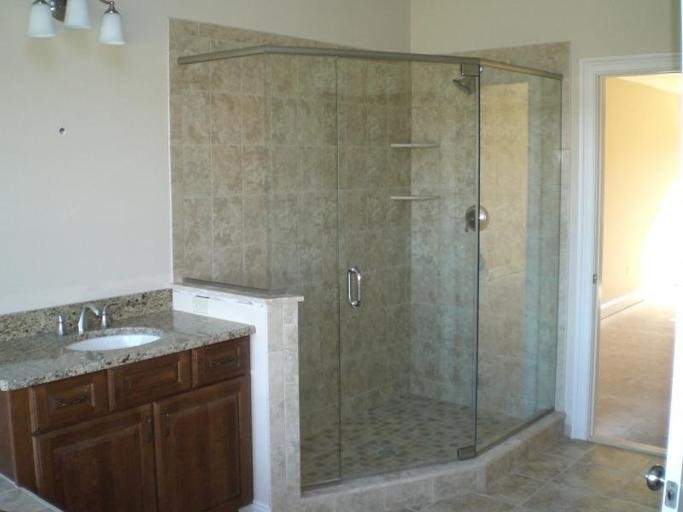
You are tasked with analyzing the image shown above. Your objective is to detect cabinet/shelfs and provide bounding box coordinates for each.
[1,336,254,512]
[390,143,442,200]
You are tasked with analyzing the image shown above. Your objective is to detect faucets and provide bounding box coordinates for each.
[77,305,100,334]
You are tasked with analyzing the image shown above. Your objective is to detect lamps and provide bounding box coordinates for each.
[25,1,126,45]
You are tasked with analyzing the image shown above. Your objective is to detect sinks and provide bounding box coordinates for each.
[62,327,163,350]
